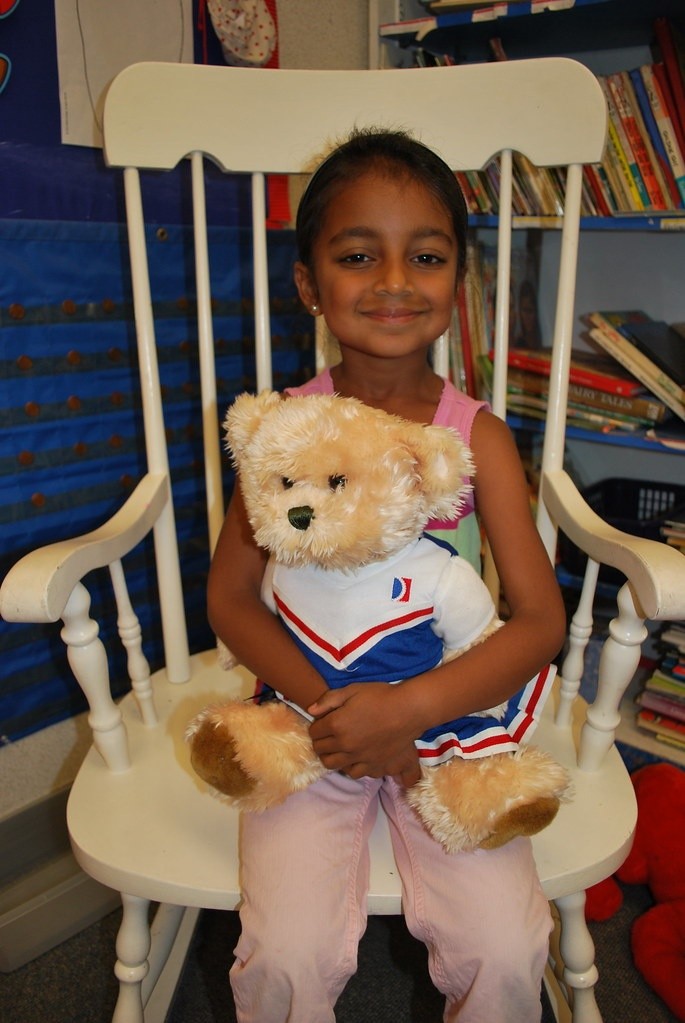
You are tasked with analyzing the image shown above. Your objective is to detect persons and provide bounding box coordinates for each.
[206,125,572,1021]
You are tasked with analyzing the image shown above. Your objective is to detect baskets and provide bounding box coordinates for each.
[568,476,684,584]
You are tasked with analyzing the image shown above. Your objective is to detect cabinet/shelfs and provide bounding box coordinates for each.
[367,0,685,769]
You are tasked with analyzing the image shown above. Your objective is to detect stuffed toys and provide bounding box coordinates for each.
[161,388,574,856]
[580,763,685,1018]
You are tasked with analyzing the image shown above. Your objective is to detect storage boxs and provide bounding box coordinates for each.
[557,478,685,575]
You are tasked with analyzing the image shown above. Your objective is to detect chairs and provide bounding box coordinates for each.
[2,58,685,1023]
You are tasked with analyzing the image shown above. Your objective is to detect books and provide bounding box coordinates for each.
[635,499,685,759]
[400,33,685,222]
[450,237,685,455]
